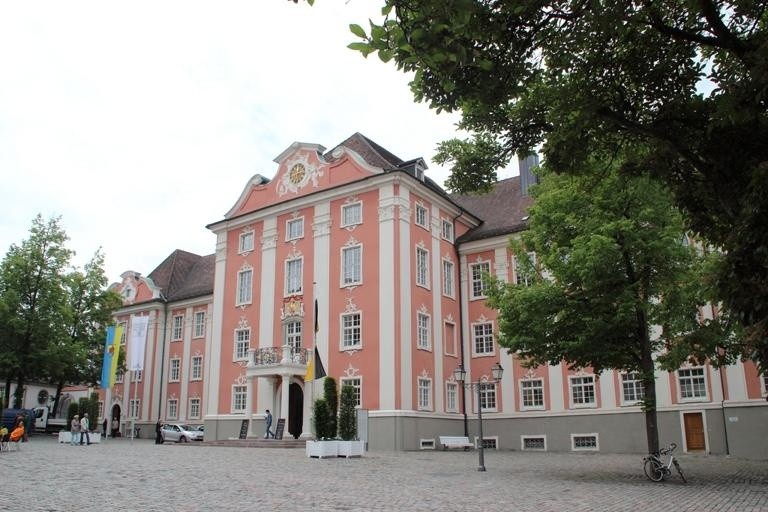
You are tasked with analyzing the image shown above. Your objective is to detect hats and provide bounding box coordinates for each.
[160,424,205,444]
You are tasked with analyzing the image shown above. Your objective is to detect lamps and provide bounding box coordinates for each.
[0,432,23,453]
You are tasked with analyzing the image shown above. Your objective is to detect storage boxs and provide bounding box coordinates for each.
[439,435,474,452]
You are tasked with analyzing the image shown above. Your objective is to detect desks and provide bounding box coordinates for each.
[452,363,504,472]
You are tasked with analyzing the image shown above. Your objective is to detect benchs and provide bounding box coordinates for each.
[315,299,319,333]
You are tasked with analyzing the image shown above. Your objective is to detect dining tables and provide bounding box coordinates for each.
[305,376,367,460]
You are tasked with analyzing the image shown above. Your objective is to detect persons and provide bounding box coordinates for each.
[112,417,119,438]
[70,414,81,446]
[103,418,107,438]
[0,412,32,442]
[156,419,164,444]
[264,410,275,439]
[80,413,92,445]
